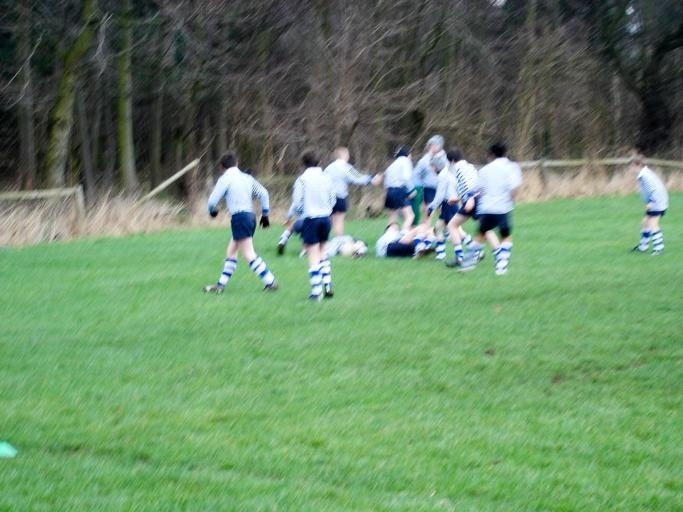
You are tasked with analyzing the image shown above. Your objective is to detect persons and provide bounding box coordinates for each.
[384,135,523,275]
[279,149,334,299]
[203,153,278,295]
[626,157,669,257]
[324,146,370,237]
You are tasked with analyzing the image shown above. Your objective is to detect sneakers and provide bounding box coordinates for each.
[262,281,277,292]
[203,284,225,293]
[278,244,284,255]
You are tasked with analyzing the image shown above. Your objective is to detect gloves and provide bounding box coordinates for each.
[260,214,270,227]
